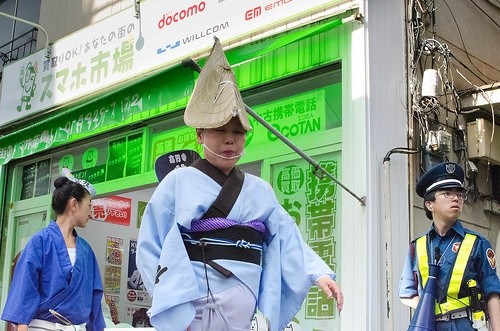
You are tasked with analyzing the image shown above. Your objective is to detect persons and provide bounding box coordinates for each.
[1,177,106,331]
[398,163,500,331]
[129,270,146,290]
[136,116,344,331]
[132,308,153,328]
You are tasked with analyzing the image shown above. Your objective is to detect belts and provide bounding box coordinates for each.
[436,309,474,321]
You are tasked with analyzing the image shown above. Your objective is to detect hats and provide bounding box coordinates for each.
[416,162,468,197]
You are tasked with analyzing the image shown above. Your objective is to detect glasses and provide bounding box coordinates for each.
[435,191,467,201]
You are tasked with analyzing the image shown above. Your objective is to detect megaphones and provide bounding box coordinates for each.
[406,264,443,331]
